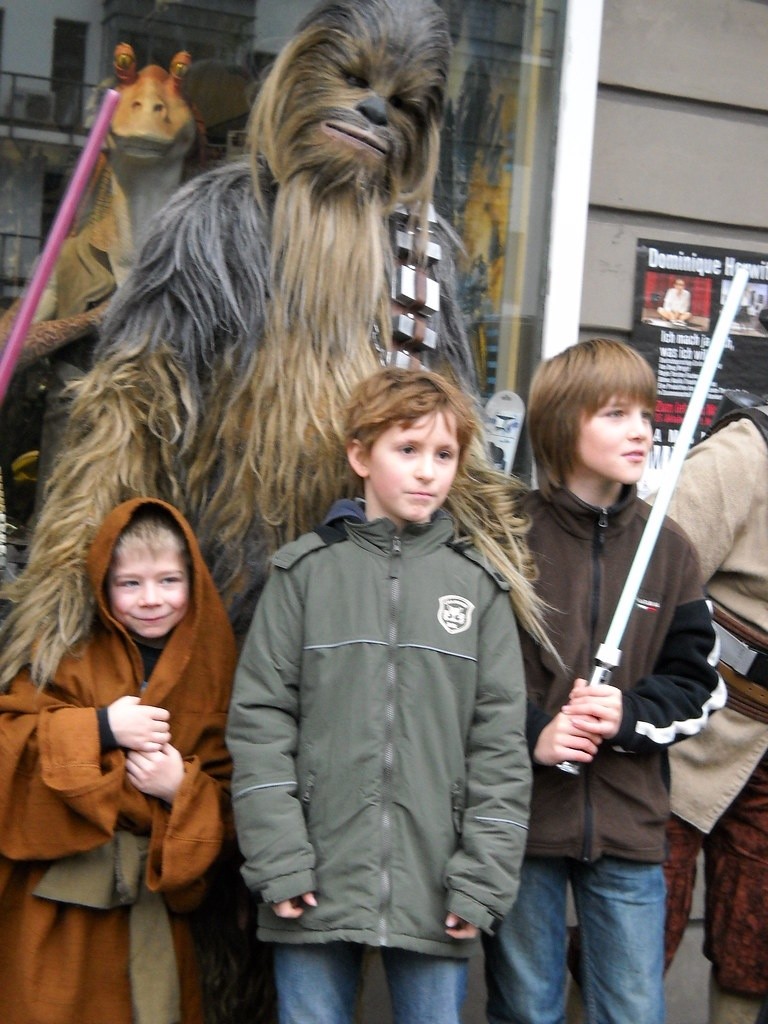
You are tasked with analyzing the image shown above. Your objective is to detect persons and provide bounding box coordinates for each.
[224,366,533,1024]
[568,304,768,1024]
[0,497,238,1024]
[656,278,693,327]
[475,338,728,1024]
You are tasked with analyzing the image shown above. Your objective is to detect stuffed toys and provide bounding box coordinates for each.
[0,0,535,1024]
